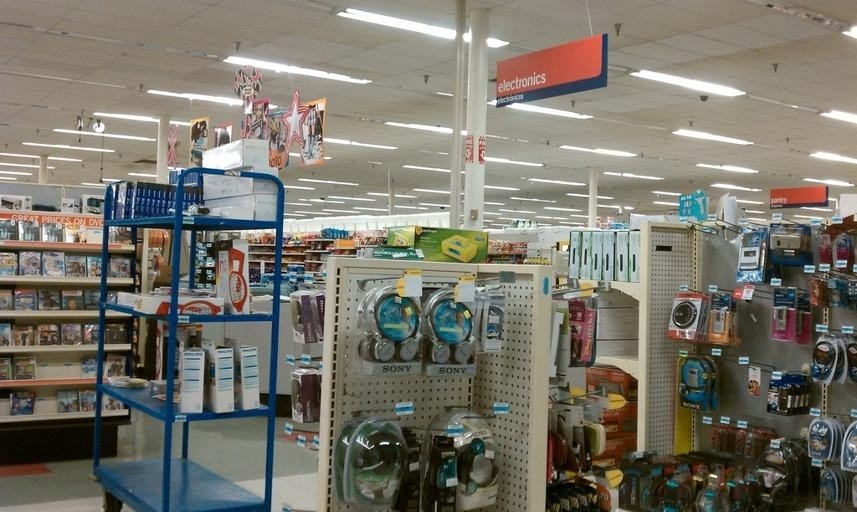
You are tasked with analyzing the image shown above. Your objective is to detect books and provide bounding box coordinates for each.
[0,357,38,380]
[0,252,131,279]
[57,389,124,413]
[10,390,36,415]
[0,289,101,310]
[0,324,128,346]
[82,354,127,378]
[0,219,131,244]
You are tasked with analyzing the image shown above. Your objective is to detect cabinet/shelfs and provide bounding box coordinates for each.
[306,236,341,282]
[567,222,697,482]
[280,241,308,280]
[0,209,138,459]
[245,242,276,285]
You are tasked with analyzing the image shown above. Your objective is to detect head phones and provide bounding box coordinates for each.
[422,288,476,365]
[334,416,408,508]
[807,335,857,385]
[354,284,421,363]
[419,411,502,509]
[616,452,756,512]
[674,353,723,414]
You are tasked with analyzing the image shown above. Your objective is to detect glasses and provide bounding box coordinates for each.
[256,108,262,111]
